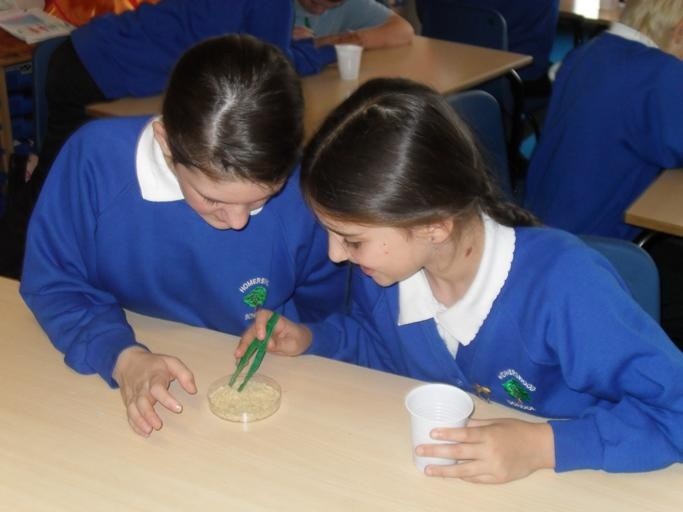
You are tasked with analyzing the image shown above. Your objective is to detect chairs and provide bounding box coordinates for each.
[448,88,516,203]
[577,232,661,334]
[7,35,84,188]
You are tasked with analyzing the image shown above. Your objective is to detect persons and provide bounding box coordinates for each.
[17,32,352,439]
[292,0,415,48]
[234,76,683,485]
[522,1,683,352]
[8,2,364,214]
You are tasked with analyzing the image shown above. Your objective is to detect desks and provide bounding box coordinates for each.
[0,276,683,511]
[0,8,69,179]
[625,169,683,241]
[86,35,533,177]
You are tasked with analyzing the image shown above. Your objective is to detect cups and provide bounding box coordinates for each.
[405,383,474,475]
[335,43,364,81]
